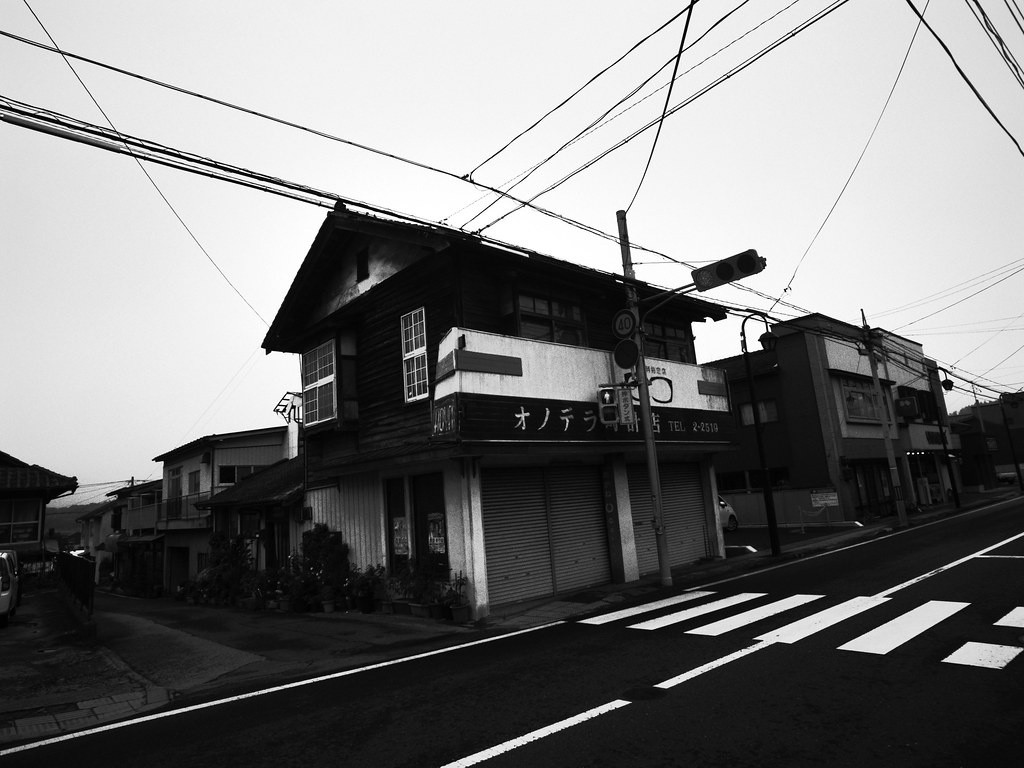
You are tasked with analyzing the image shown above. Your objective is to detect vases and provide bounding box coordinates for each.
[279,597,293,612]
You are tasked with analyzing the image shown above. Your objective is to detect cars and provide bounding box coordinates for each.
[0,551,19,616]
[718,495,736,532]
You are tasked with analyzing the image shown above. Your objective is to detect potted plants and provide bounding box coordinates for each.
[321,591,337,613]
[350,564,385,613]
[381,548,471,624]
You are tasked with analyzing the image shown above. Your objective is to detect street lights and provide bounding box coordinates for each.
[929,368,960,509]
[739,314,782,559]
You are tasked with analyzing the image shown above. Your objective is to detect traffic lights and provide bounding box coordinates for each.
[597,388,621,425]
[691,248,766,293]
